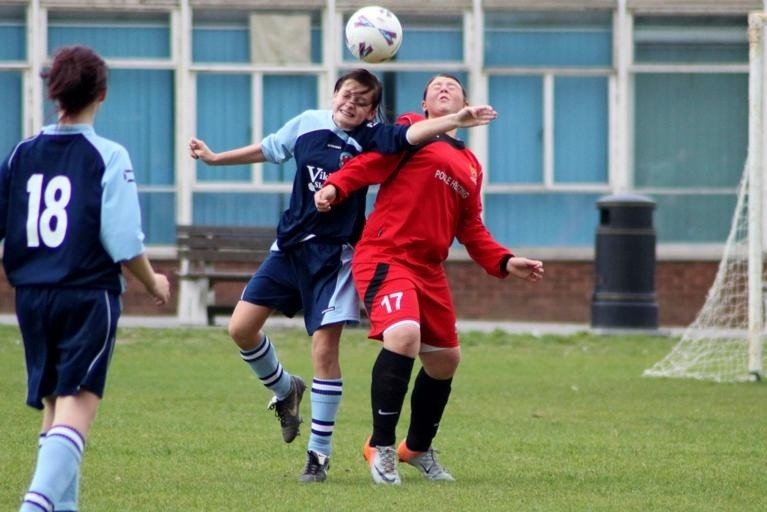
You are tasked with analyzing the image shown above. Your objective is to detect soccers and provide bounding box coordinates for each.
[345,6,402,62]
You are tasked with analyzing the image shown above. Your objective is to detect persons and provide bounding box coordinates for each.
[0,42,172,512]
[312,71,545,484]
[188,67,499,484]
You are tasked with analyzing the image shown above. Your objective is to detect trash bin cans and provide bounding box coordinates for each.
[589,192,659,329]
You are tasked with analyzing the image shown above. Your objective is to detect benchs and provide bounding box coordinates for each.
[172,223,279,327]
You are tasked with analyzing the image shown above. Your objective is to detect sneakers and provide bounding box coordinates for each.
[267,376,305,443]
[301,450,331,483]
[363,435,401,486]
[398,438,454,480]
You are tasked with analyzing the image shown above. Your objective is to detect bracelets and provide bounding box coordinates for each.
[501,254,514,273]
